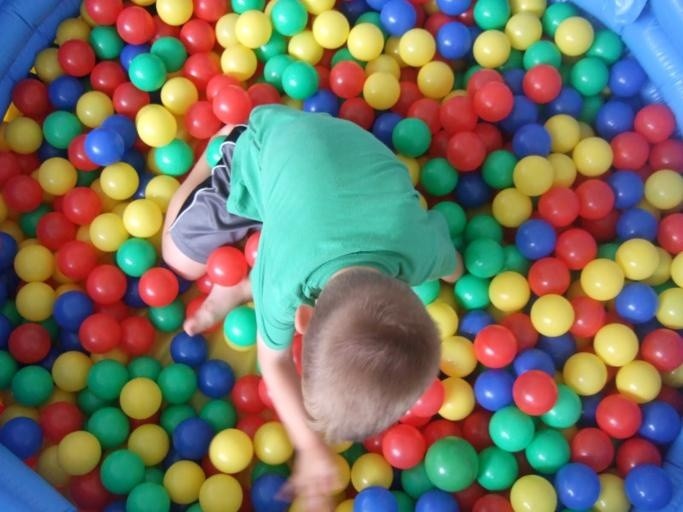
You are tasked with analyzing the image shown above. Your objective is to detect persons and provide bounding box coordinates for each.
[161,103,464,512]
